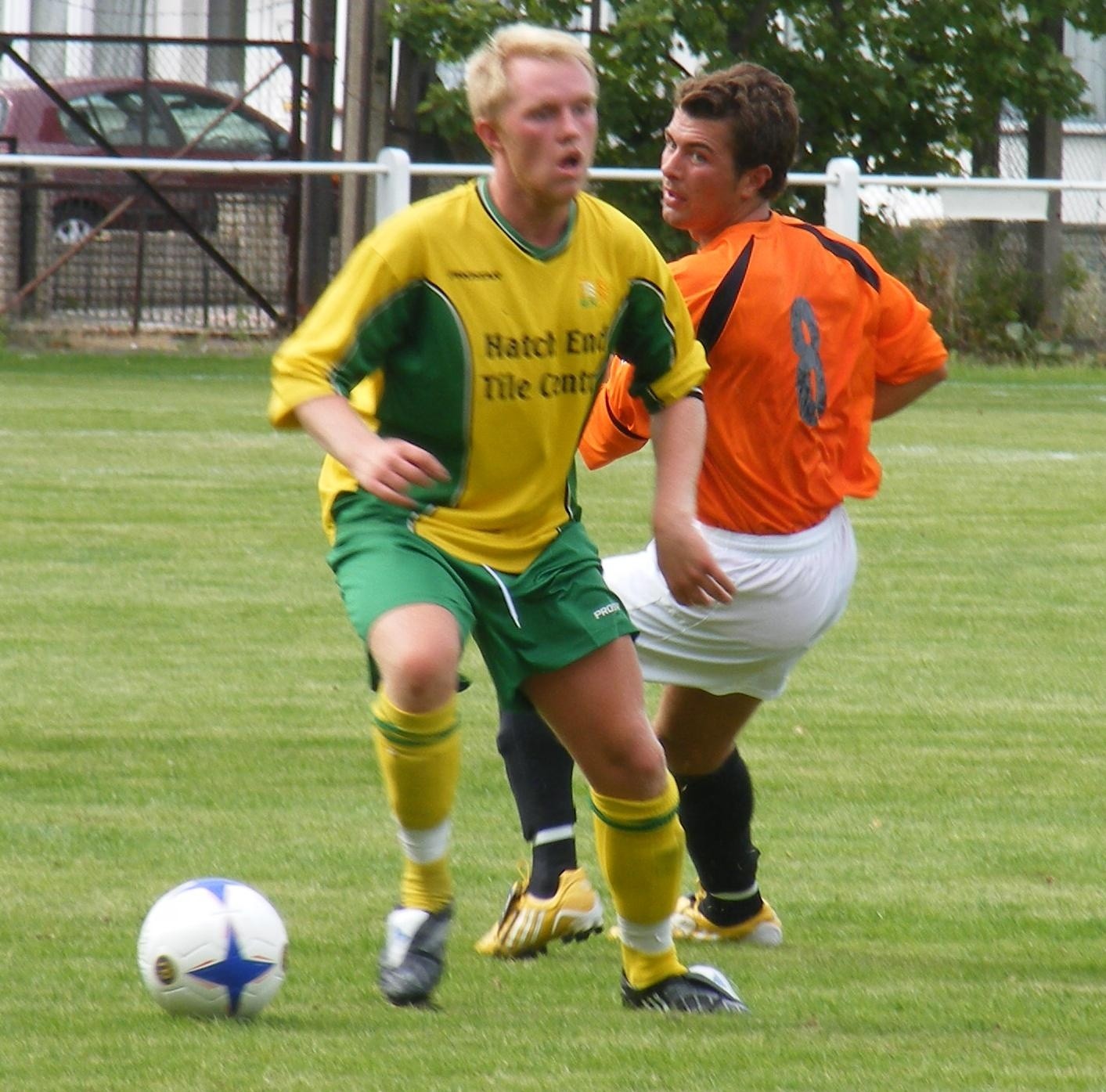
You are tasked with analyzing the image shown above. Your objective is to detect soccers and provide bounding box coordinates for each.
[135,876,292,1020]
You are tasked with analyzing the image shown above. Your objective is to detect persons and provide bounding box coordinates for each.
[472,63,949,958]
[265,22,746,1018]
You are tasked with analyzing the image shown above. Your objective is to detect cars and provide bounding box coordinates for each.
[0,75,369,317]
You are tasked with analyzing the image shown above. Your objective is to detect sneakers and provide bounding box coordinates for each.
[618,964,751,1016]
[379,905,456,1004]
[607,878,784,947]
[473,860,605,962]
